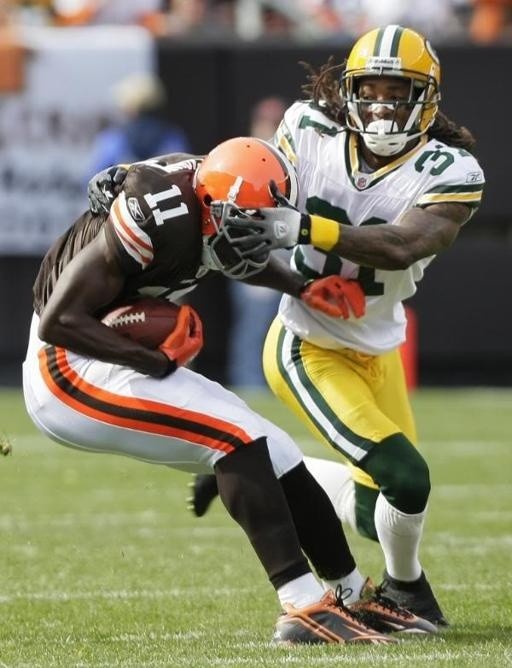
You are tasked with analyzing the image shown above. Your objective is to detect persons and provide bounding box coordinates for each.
[0,0,512,384]
[21,136,439,647]
[87,23,487,626]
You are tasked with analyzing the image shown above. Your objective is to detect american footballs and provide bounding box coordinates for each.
[100,301,193,346]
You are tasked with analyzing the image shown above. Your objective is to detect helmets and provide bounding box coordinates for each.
[192,136,300,235]
[345,24,441,92]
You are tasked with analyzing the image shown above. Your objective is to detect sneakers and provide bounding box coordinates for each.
[186,473,218,517]
[269,569,449,644]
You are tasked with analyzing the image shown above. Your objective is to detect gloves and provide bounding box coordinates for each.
[158,304,203,368]
[87,164,130,214]
[298,274,366,320]
[225,178,311,259]
